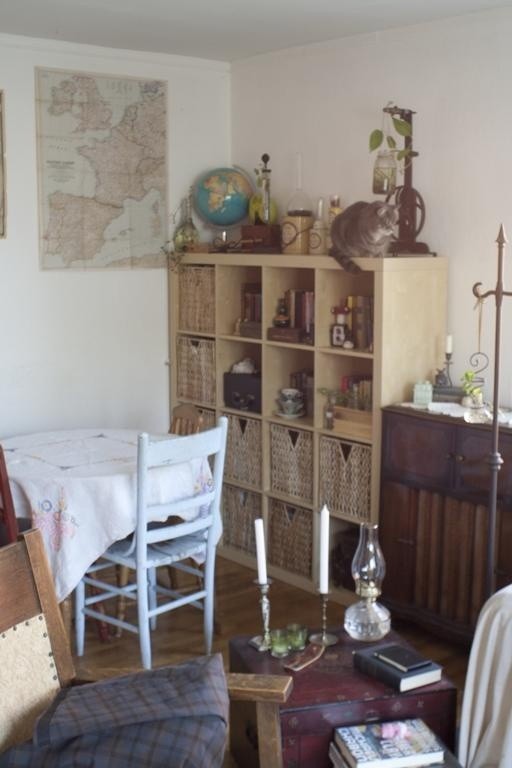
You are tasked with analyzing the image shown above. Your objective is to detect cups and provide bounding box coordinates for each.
[285,623,308,652]
[276,398,305,414]
[269,629,290,659]
[281,388,303,400]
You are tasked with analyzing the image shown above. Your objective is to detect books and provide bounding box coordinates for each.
[353,643,442,692]
[345,295,373,354]
[290,371,313,418]
[243,282,260,323]
[286,288,314,343]
[342,374,371,407]
[327,718,446,768]
[373,644,432,673]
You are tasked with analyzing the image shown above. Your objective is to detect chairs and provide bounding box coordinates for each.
[0,528,294,768]
[75,416,229,671]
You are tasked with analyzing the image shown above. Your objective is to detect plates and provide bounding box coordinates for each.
[273,411,307,420]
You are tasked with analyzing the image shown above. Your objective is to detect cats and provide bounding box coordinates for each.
[328,201,402,275]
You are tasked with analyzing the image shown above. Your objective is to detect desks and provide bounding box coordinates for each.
[229,626,457,768]
[0,429,223,642]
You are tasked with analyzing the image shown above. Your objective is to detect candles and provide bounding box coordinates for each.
[444,335,452,354]
[319,503,329,594]
[254,518,268,584]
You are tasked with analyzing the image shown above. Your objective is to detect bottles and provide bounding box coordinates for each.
[172,193,343,255]
[413,379,433,409]
[322,396,335,431]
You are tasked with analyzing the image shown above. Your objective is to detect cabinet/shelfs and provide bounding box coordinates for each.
[168,248,448,607]
[379,402,512,647]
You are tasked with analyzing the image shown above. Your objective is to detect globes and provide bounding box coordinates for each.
[193,164,256,252]
